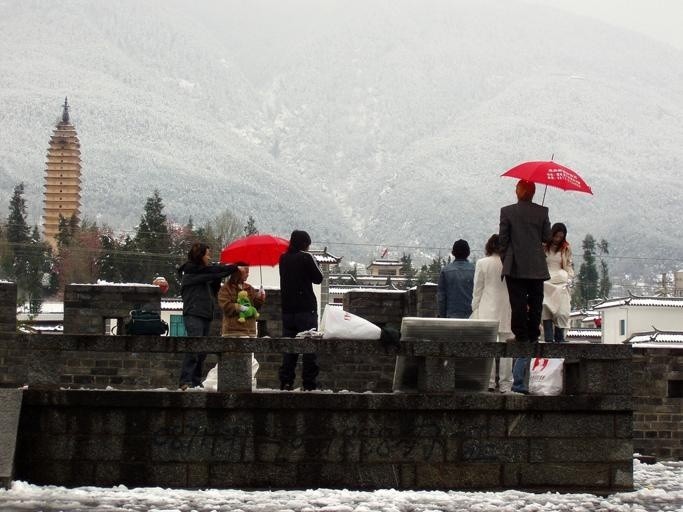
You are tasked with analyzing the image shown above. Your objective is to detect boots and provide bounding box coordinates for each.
[543,319,569,342]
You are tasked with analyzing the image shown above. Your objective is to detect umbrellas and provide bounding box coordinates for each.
[218,231,290,294]
[499,153,596,209]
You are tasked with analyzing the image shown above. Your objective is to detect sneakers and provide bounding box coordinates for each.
[506,336,530,344]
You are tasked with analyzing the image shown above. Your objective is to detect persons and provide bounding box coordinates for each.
[494,179,554,343]
[216,264,267,339]
[511,358,532,395]
[434,234,475,319]
[466,230,514,394]
[541,220,578,344]
[278,228,326,393]
[593,318,601,329]
[174,241,249,391]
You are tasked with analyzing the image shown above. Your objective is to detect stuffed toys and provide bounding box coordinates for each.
[235,289,262,324]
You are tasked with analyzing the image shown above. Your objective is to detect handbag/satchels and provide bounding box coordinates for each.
[129,309,170,335]
